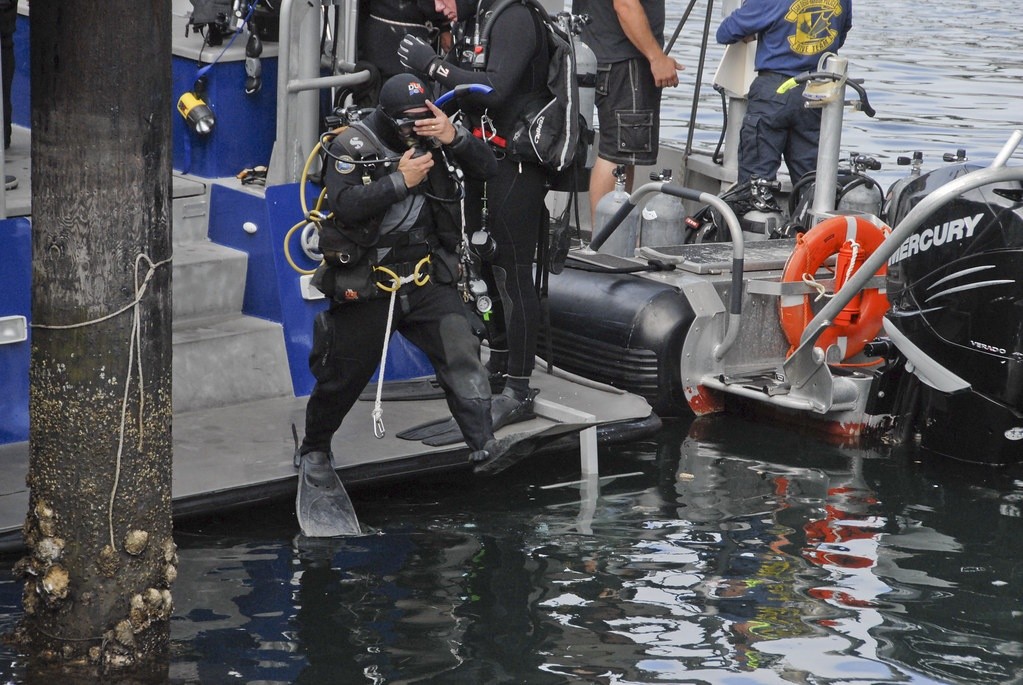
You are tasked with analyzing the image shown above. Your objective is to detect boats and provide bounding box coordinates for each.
[534,0,1023,482]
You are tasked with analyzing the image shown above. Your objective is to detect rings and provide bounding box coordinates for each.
[432,125,435,130]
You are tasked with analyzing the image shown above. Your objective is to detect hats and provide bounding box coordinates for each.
[379,73,432,118]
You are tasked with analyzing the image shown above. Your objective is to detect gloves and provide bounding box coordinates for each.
[397,34,443,76]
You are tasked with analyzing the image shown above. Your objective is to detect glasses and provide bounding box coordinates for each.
[393,110,436,137]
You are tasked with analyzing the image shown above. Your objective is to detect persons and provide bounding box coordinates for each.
[0,0,19,191]
[295,1,604,537]
[716,0,852,230]
[572,0,686,244]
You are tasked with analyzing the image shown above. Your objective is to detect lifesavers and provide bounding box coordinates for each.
[778,215,900,361]
